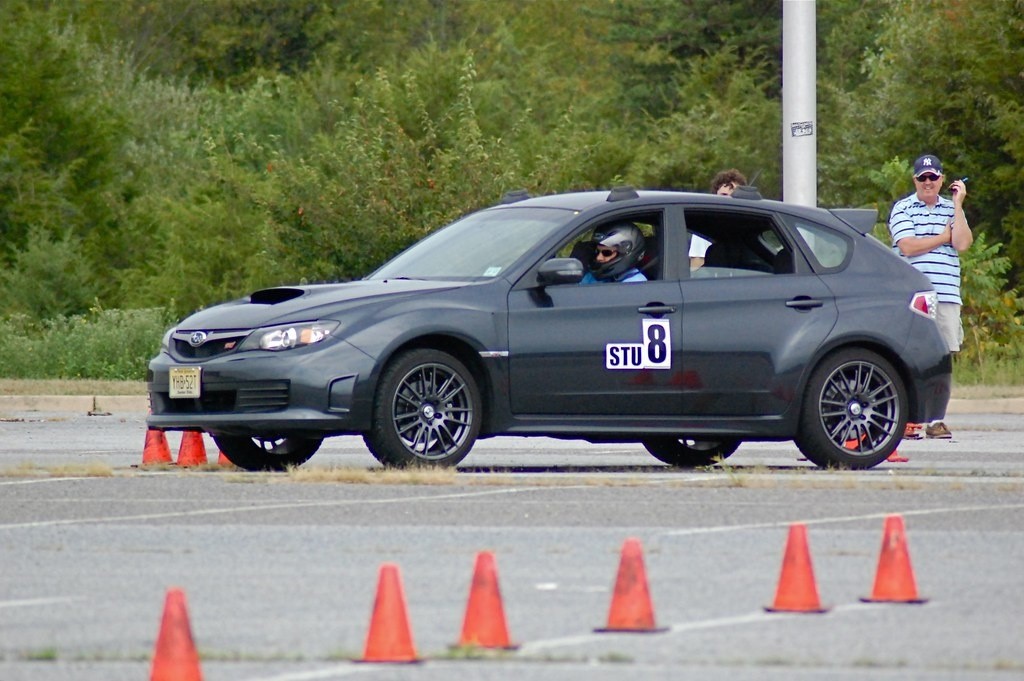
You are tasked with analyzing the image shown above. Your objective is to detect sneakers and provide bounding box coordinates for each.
[926,423,952,439]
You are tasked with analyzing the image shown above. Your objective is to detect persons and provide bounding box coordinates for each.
[578,220,648,284]
[688,168,748,272]
[887,154,973,440]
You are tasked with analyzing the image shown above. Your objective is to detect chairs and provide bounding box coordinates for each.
[642,229,693,278]
[570,224,613,271]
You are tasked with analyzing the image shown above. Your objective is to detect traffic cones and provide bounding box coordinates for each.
[130,409,176,467]
[217,448,233,466]
[591,537,671,634]
[151,589,205,681]
[763,522,830,613]
[845,435,908,462]
[445,552,520,651]
[350,563,424,665]
[169,430,208,468]
[858,514,928,604]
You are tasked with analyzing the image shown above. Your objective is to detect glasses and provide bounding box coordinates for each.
[916,173,940,182]
[595,249,617,257]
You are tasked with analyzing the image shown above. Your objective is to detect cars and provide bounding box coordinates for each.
[145,186,952,472]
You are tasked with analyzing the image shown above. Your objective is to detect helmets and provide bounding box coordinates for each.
[587,221,645,281]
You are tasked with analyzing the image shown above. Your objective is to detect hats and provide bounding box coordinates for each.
[914,155,943,178]
